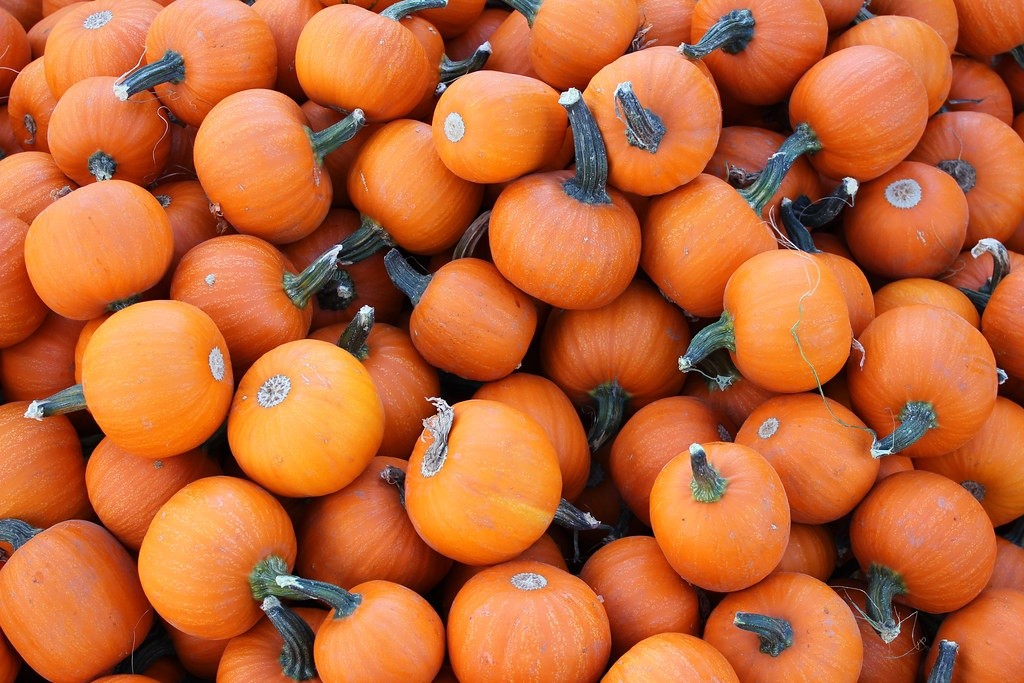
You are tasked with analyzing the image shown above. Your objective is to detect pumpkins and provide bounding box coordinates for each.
[0,0,1023,683]
